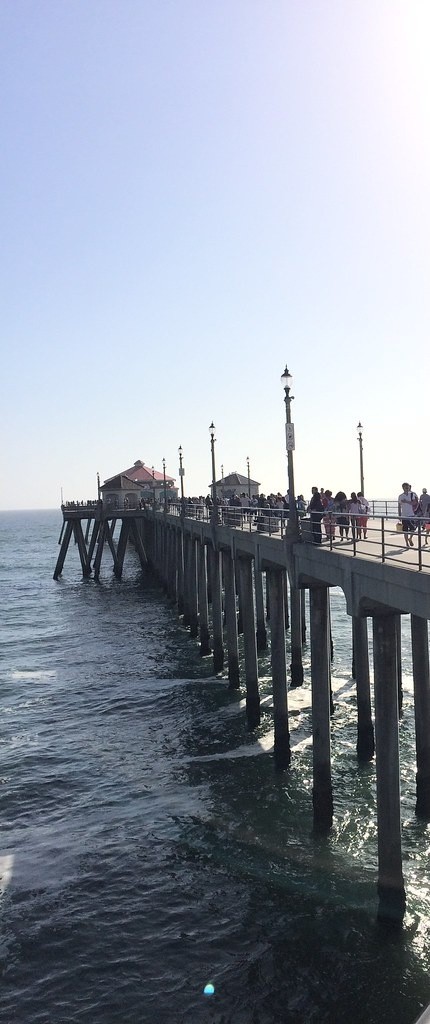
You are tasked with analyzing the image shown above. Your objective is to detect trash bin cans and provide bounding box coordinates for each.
[301,518,314,542]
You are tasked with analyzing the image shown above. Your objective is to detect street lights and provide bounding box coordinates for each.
[280,363,303,544]
[161,458,169,513]
[208,420,222,526]
[356,420,365,499]
[220,463,224,507]
[178,445,186,518]
[246,456,251,506]
[96,471,100,500]
[151,464,156,510]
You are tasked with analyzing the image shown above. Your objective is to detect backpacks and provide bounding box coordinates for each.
[359,504,367,514]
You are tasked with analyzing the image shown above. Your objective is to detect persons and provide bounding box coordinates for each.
[397,483,430,548]
[66,486,369,542]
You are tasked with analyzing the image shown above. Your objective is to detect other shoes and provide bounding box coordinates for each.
[408,538,413,546]
[327,534,368,541]
[406,544,411,548]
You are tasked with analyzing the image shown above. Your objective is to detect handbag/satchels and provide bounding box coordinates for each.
[411,492,421,514]
[336,517,346,524]
[396,521,403,532]
